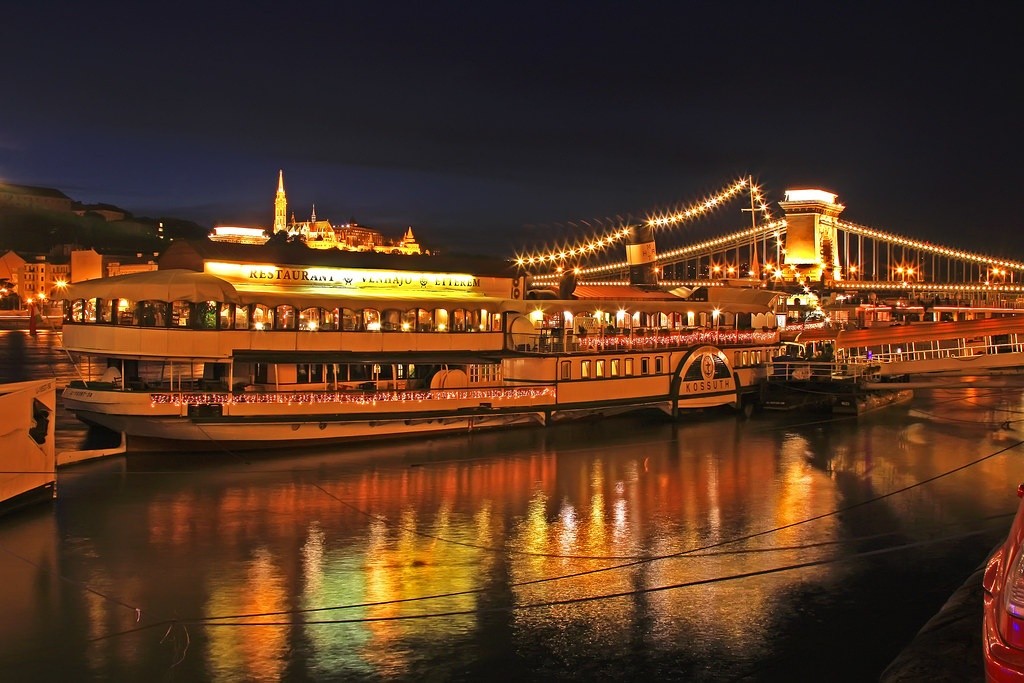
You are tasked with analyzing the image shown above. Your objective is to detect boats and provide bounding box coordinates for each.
[54,267,782,454]
